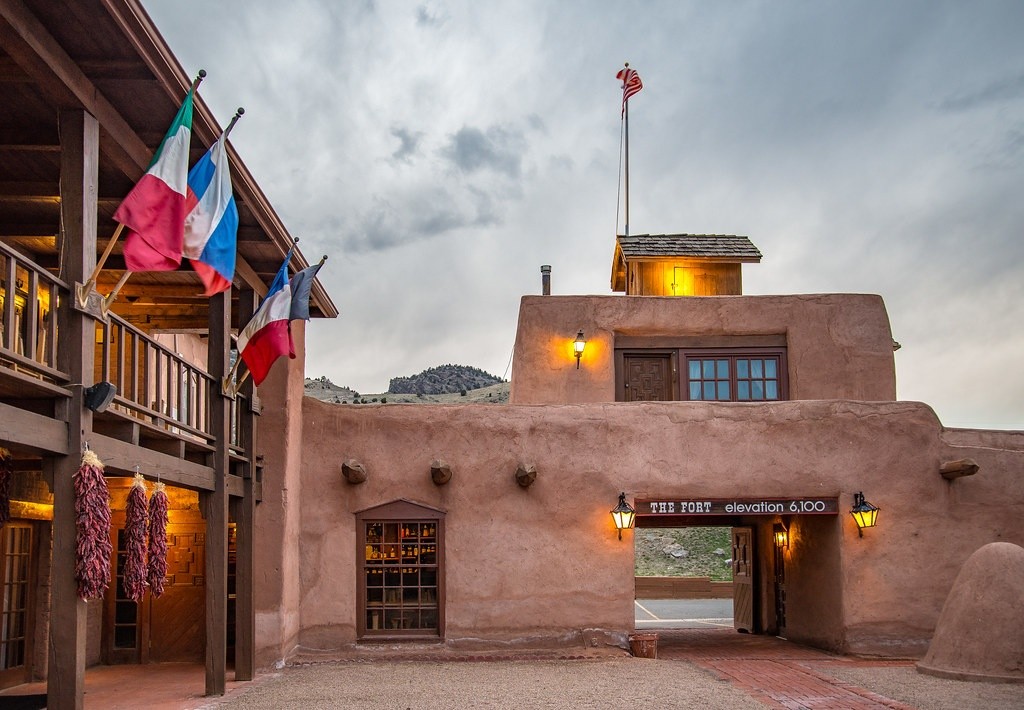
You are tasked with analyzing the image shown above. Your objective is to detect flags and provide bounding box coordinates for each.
[235,249,298,389]
[180,127,240,299]
[288,264,322,323]
[616,67,643,121]
[112,82,193,272]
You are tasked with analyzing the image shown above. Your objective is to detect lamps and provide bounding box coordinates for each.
[609,492,639,541]
[85,381,117,412]
[849,491,880,538]
[772,523,789,550]
[573,330,587,370]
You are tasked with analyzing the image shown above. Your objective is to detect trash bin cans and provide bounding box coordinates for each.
[628,633,659,659]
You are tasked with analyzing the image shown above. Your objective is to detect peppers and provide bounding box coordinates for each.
[72,463,114,602]
[148,491,170,599]
[123,484,149,604]
